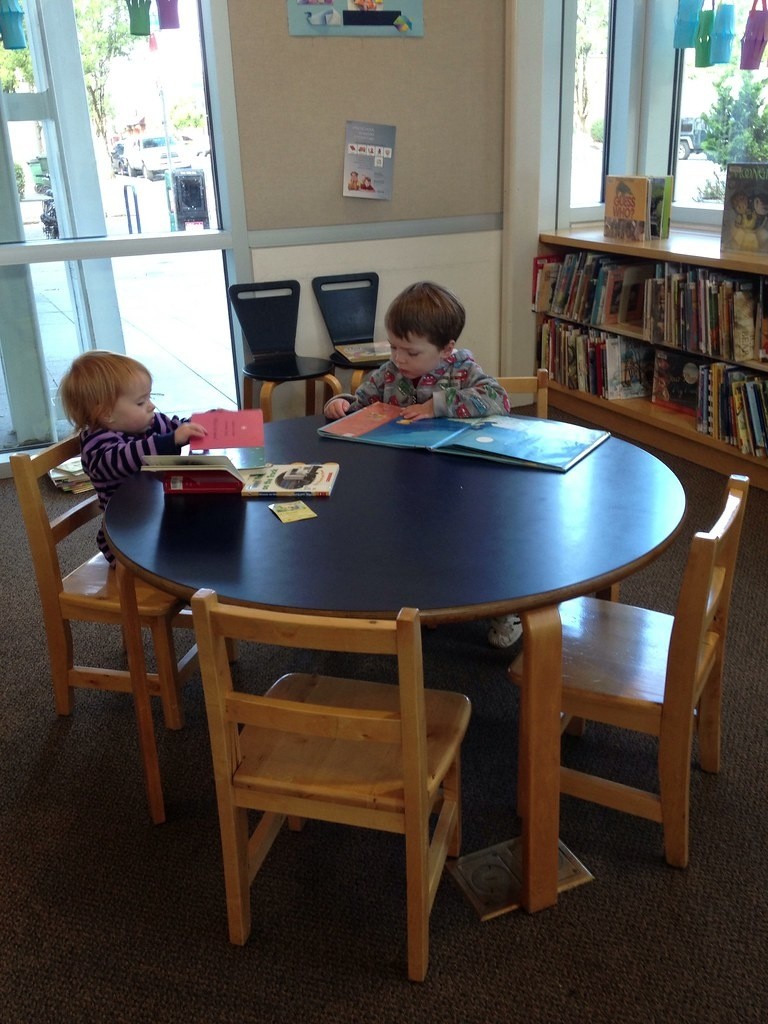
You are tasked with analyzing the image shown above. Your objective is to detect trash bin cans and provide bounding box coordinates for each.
[26,156,52,194]
[170,168,210,231]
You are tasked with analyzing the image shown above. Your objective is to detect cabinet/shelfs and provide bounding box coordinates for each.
[534,222,768,490]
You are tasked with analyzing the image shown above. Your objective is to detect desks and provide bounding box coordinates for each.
[102,414,687,914]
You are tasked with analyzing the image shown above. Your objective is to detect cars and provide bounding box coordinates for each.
[183,131,211,158]
[110,134,190,183]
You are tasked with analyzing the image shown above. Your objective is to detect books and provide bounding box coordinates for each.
[48,456,96,494]
[241,461,340,496]
[140,455,266,495]
[317,400,612,473]
[604,174,652,243]
[649,175,674,239]
[190,407,265,448]
[529,253,768,457]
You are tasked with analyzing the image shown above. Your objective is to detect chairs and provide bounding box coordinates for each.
[228,280,342,423]
[190,589,472,981]
[493,368,548,420]
[9,431,237,731]
[311,272,389,414]
[506,473,749,867]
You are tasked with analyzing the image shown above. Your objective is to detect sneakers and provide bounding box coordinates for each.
[488,612,523,648]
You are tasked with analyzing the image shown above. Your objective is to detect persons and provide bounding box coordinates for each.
[720,164,768,256]
[360,176,375,190]
[322,281,524,649]
[348,171,360,190]
[54,351,209,570]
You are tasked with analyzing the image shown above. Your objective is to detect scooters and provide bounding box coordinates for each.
[34,173,58,239]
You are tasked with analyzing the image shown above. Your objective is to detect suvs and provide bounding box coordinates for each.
[677,118,710,160]
